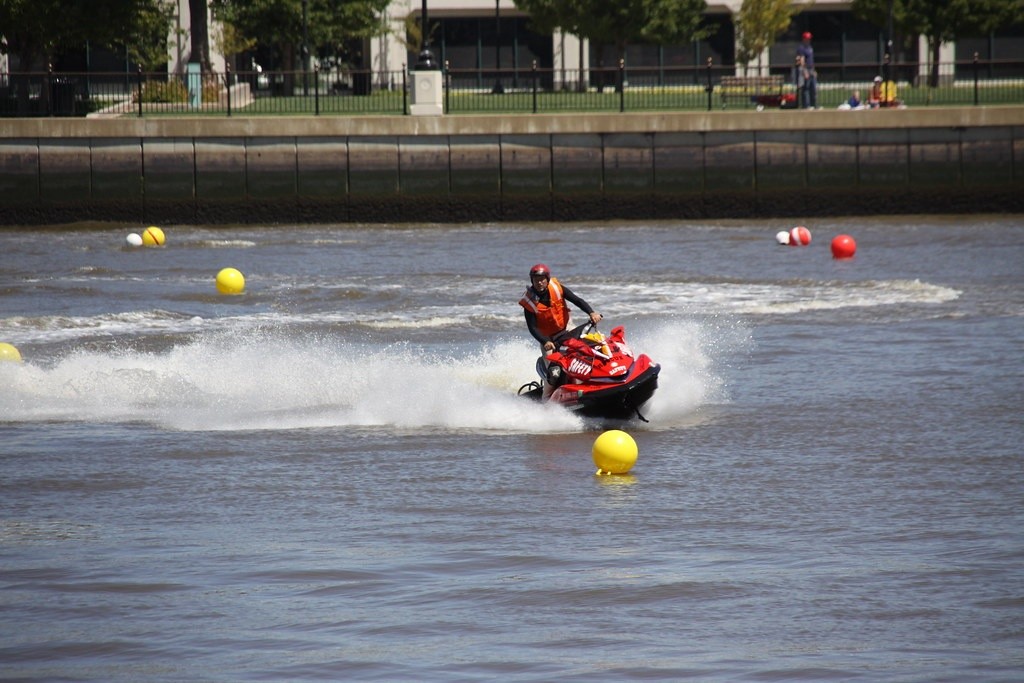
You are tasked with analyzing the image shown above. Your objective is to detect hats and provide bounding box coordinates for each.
[874,76,883,81]
[803,32,812,38]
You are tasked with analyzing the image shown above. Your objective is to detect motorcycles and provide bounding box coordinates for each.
[517,314,664,422]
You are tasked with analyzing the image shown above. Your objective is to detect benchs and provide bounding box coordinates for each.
[720,74,784,111]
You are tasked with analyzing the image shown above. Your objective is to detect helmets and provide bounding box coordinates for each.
[530,264,551,280]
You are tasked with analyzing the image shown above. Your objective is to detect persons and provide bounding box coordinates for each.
[840,76,903,107]
[518,263,602,400]
[795,33,819,110]
